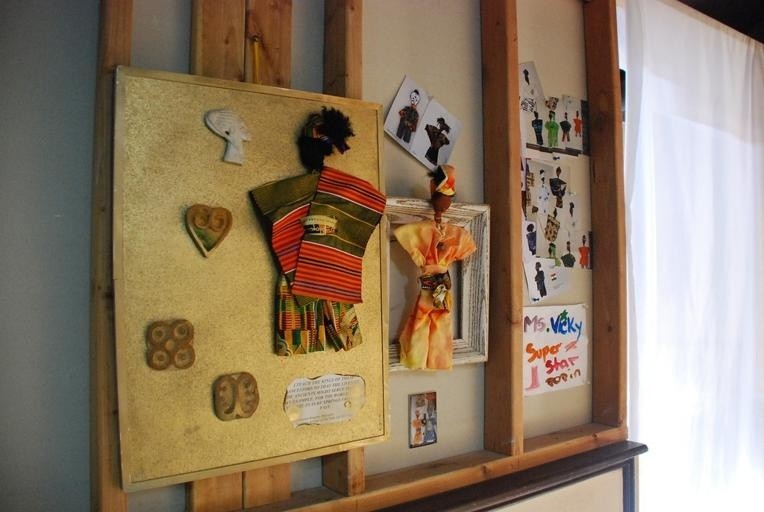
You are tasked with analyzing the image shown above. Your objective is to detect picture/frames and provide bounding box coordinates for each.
[386,196,491,374]
